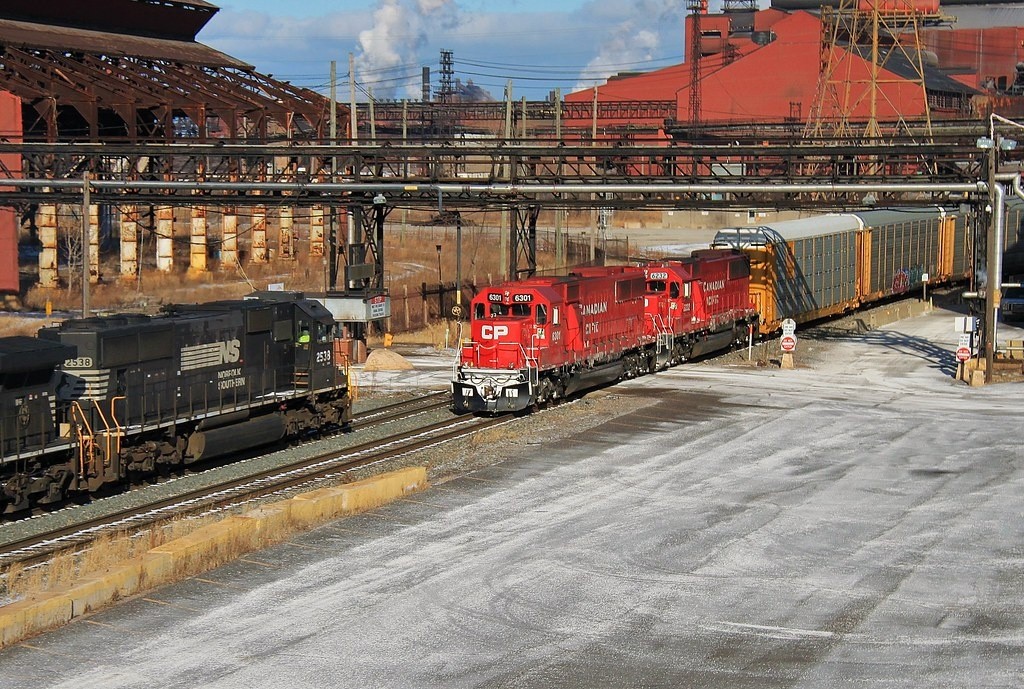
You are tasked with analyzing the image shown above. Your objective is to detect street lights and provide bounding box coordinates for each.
[976,136,1017,352]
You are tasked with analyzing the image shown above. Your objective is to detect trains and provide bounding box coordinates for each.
[449,197,1024,418]
[0,294,359,519]
